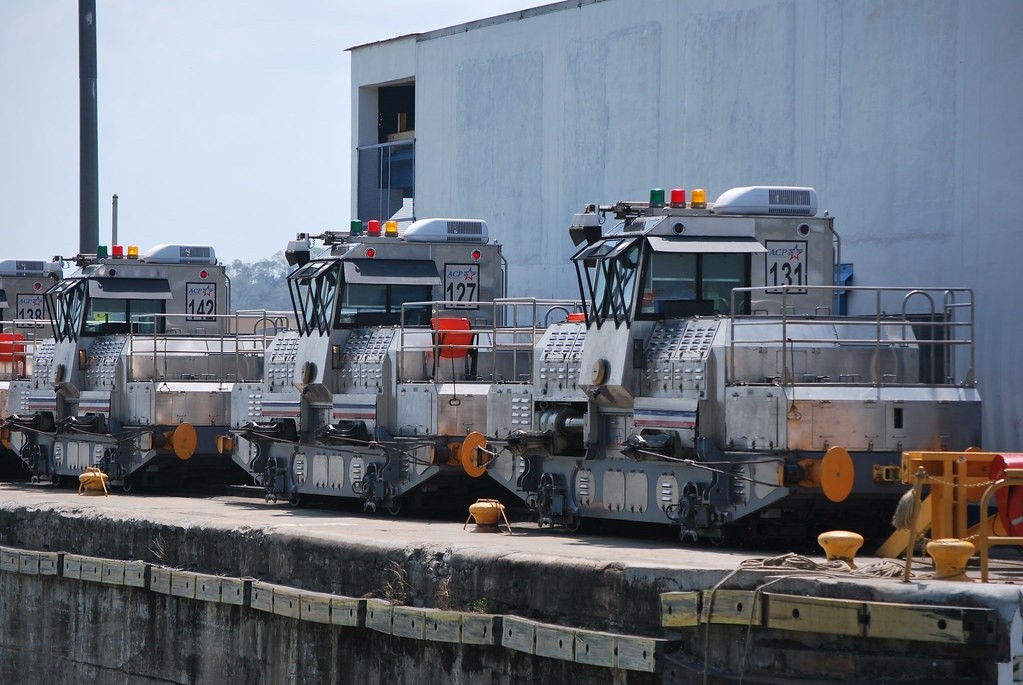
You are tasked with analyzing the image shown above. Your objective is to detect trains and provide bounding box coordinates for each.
[0,184,984,555]
